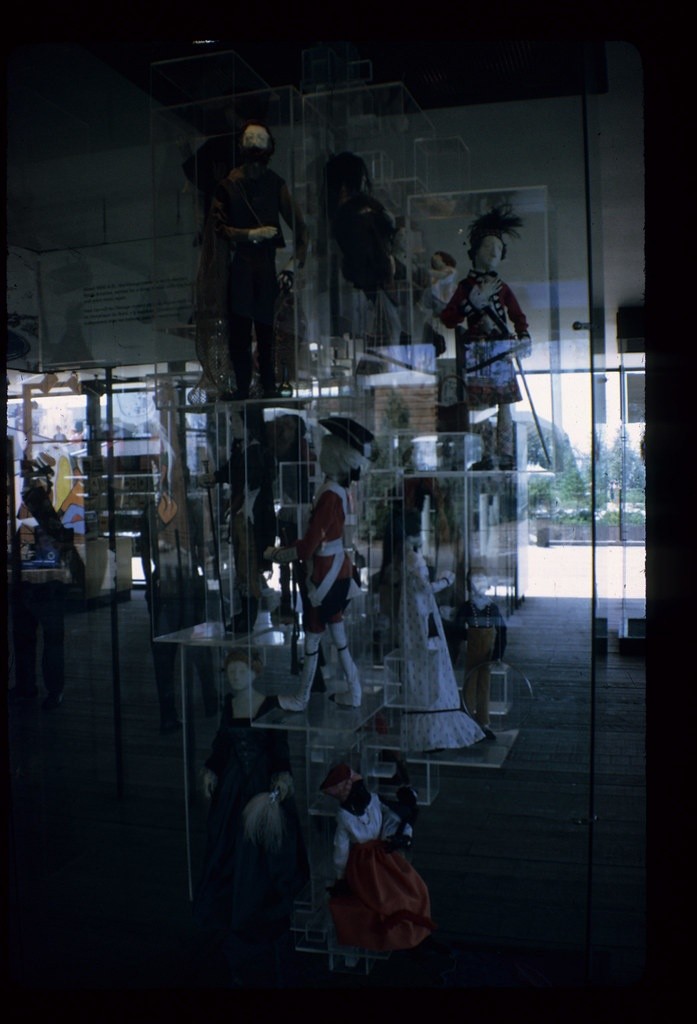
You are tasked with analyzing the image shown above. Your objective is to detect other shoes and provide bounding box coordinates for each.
[41,689,64,710]
[8,685,38,704]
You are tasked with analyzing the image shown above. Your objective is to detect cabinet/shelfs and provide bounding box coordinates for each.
[151,81,558,973]
[81,471,163,532]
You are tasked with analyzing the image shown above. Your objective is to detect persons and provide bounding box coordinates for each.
[319,763,437,950]
[199,402,307,632]
[378,446,451,591]
[210,121,406,401]
[278,417,377,711]
[413,203,531,470]
[401,538,486,752]
[200,650,311,928]
[452,568,507,743]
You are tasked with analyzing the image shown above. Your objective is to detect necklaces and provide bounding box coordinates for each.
[352,805,371,825]
